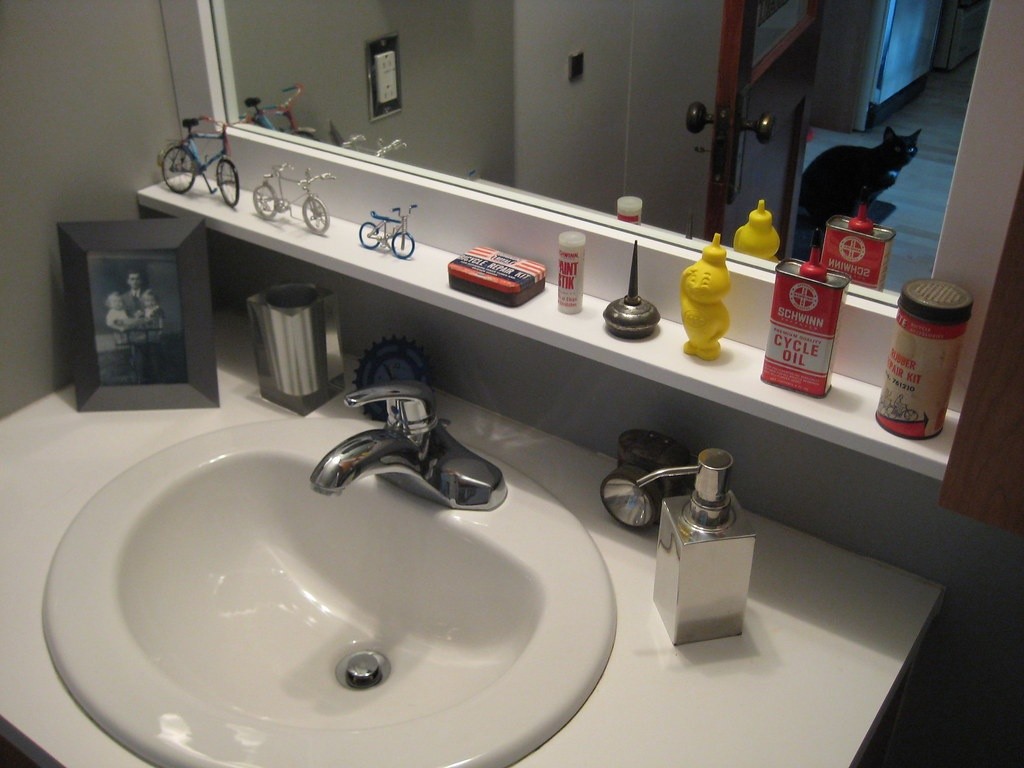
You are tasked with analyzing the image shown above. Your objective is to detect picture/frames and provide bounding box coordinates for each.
[55,218,222,416]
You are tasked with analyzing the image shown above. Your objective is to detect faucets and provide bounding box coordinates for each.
[309,379,509,513]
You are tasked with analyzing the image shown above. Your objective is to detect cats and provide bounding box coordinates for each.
[799,126,922,231]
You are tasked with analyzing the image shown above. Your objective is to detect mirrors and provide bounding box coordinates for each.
[212,1,989,296]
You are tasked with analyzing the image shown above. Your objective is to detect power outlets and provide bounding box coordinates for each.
[366,32,403,123]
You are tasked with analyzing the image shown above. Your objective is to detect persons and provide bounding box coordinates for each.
[679,232,729,360]
[734,199,780,263]
[88,250,189,385]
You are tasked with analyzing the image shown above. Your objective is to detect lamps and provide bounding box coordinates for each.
[601,429,692,529]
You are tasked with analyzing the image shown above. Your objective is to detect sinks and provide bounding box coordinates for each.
[40,417,617,768]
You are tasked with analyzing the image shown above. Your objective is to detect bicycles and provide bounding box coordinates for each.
[252,162,336,234]
[359,203,418,259]
[240,83,322,143]
[340,134,407,161]
[157,114,255,207]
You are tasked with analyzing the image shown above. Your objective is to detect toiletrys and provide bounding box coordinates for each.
[637,448,759,648]
